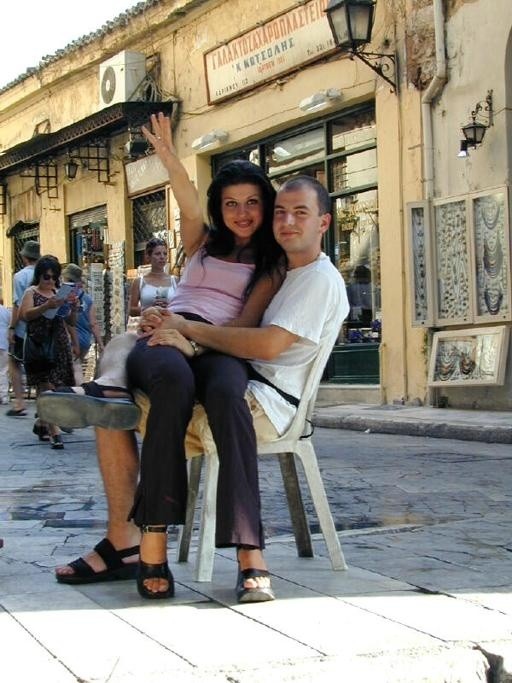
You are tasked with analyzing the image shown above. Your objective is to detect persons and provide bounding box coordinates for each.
[129,237,180,317]
[128,112,276,603]
[37,172,352,583]
[60,263,105,384]
[0,296,11,404]
[18,253,78,449]
[5,240,43,417]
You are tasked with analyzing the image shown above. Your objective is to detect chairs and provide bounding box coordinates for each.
[175,316,352,582]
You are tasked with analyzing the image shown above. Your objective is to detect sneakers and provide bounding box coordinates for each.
[2,397,9,404]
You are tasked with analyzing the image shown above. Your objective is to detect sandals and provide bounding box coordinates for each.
[7,408,27,415]
[235,544,274,602]
[135,525,175,599]
[55,538,140,584]
[36,380,141,431]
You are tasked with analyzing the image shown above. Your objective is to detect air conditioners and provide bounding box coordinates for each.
[98,48,148,109]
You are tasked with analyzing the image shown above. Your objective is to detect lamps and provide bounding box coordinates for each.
[323,0,401,97]
[191,131,231,151]
[297,86,345,113]
[65,150,88,178]
[457,88,493,158]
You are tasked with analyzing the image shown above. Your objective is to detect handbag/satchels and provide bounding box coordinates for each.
[28,332,59,369]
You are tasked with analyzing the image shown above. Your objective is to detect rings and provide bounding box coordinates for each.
[157,135,161,138]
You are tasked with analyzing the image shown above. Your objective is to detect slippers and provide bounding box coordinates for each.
[33,423,52,440]
[50,434,64,449]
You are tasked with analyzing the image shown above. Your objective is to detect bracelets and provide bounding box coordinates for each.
[190,339,197,357]
[9,325,14,328]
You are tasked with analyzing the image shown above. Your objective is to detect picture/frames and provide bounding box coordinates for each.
[406,183,512,388]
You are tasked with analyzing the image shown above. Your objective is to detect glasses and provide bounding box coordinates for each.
[153,237,165,244]
[44,273,58,280]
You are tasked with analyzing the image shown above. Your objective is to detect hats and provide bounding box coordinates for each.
[19,240,40,259]
[63,262,82,281]
